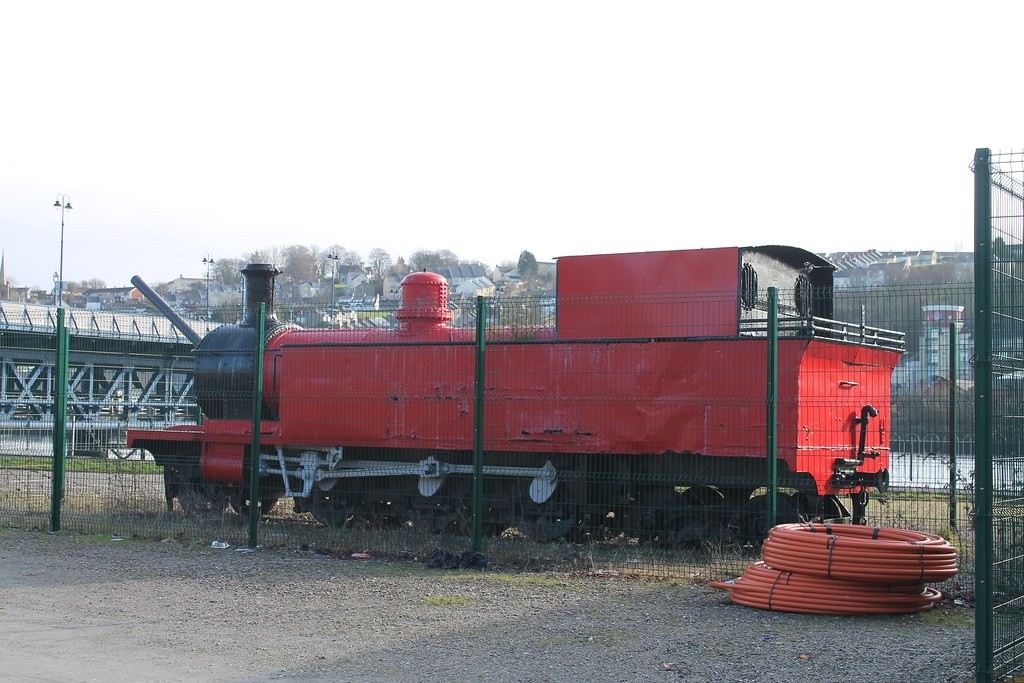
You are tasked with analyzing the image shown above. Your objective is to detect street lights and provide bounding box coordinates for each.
[327,248,341,329]
[202,253,215,322]
[389,285,399,330]
[51,194,72,307]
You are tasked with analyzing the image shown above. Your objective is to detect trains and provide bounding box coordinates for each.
[124,263,907,553]
